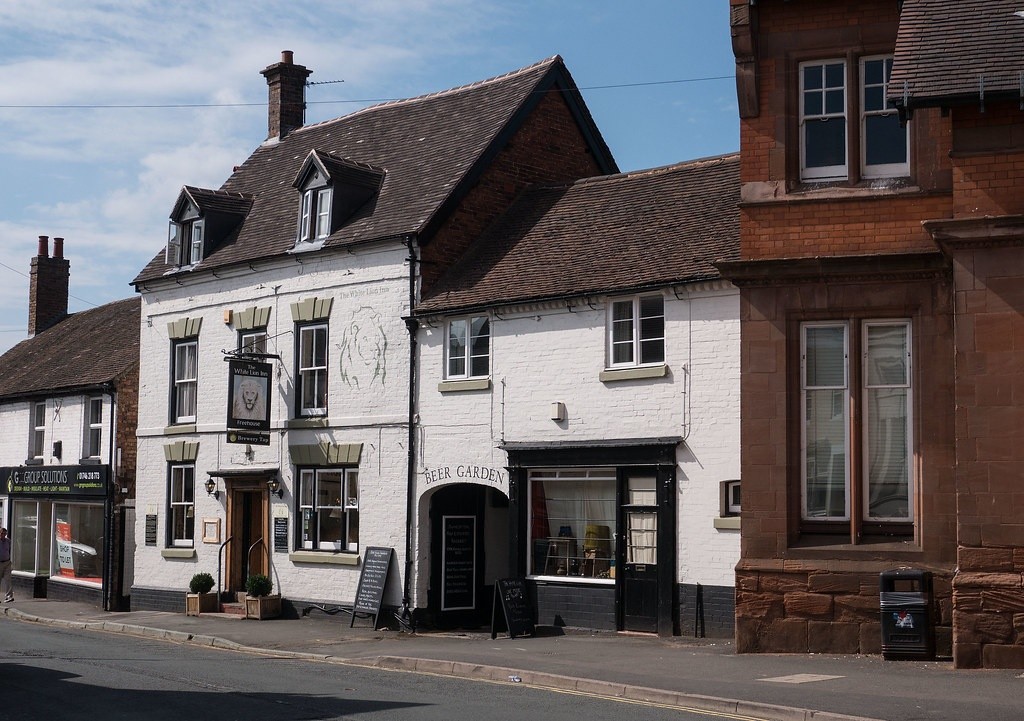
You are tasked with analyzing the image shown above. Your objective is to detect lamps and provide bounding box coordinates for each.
[204,477,219,500]
[267,477,284,500]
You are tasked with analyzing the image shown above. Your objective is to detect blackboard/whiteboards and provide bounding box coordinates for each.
[353,546,394,614]
[492,577,534,629]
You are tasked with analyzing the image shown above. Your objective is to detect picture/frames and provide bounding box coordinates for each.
[201,517,220,544]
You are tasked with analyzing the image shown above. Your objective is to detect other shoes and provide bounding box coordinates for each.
[4,597,14,602]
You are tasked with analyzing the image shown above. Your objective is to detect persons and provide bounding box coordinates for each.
[0,528,14,603]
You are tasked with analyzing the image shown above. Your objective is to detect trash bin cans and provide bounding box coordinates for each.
[879,569,936,661]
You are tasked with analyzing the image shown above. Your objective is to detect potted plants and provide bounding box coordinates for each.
[244,574,281,620]
[185,572,218,618]
